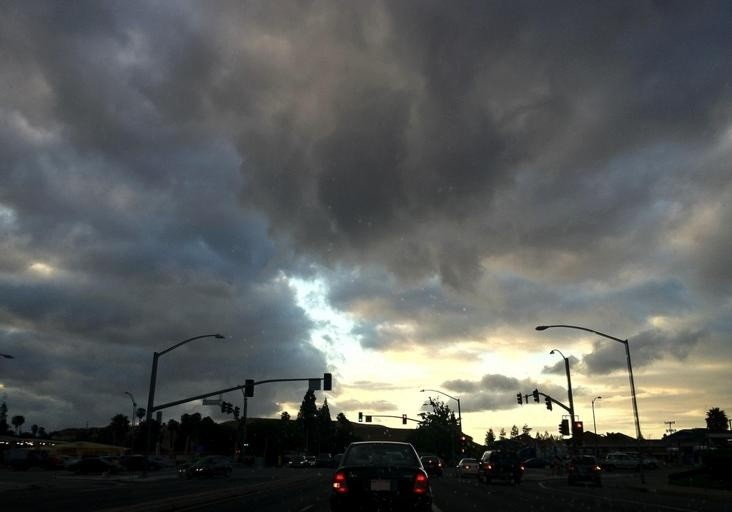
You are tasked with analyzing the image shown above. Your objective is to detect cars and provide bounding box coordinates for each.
[421,455,443,477]
[455,457,480,479]
[328,440,433,512]
[176,455,233,479]
[478,449,526,485]
[567,454,603,487]
[605,452,640,471]
[288,455,320,468]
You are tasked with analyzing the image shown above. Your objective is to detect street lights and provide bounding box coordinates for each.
[125,391,137,426]
[146,332,226,421]
[535,324,643,441]
[420,389,462,432]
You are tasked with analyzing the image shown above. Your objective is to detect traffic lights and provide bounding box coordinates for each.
[574,421,583,439]
[533,388,540,403]
[545,396,552,411]
[358,412,363,422]
[221,401,227,413]
[558,419,569,436]
[227,402,233,414]
[402,414,407,425]
[234,406,240,417]
[517,393,522,405]
